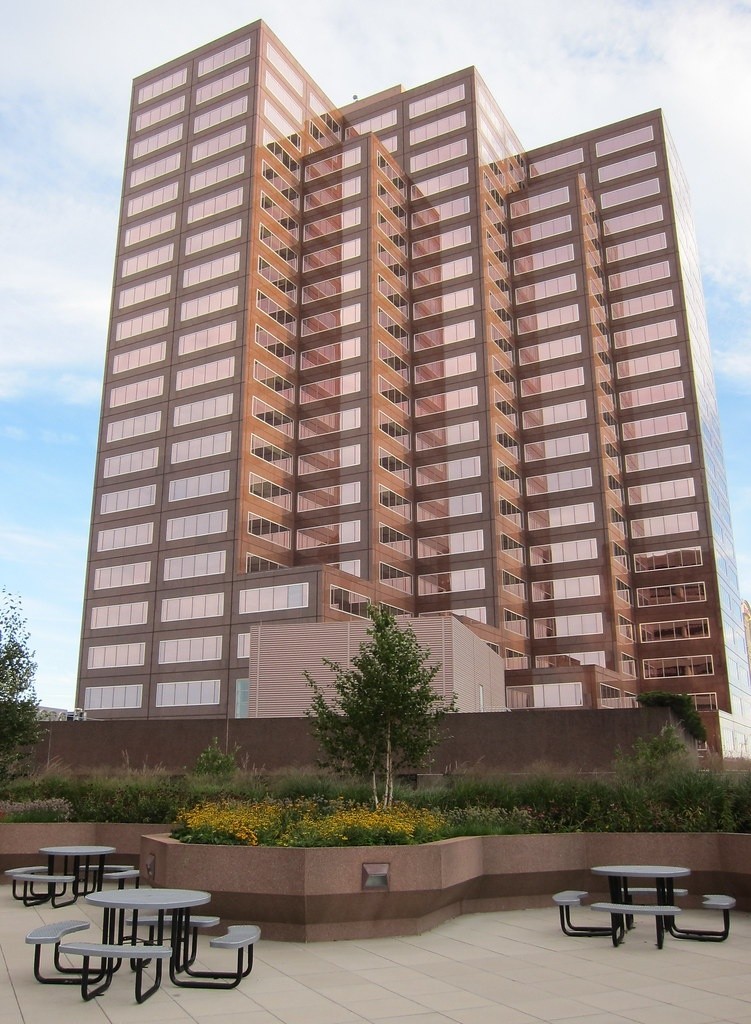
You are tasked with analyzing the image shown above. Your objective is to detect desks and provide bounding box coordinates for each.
[84,888,212,989]
[37,845,117,907]
[591,865,691,937]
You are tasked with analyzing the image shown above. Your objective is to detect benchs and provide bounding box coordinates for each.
[12,874,76,883]
[24,920,91,945]
[4,866,48,876]
[621,887,688,897]
[552,890,589,905]
[702,894,737,909]
[210,925,262,949]
[591,902,682,917]
[60,941,173,959]
[79,864,134,870]
[103,870,140,879]
[126,914,220,929]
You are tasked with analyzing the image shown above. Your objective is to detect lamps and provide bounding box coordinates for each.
[144,854,155,880]
[363,862,390,890]
